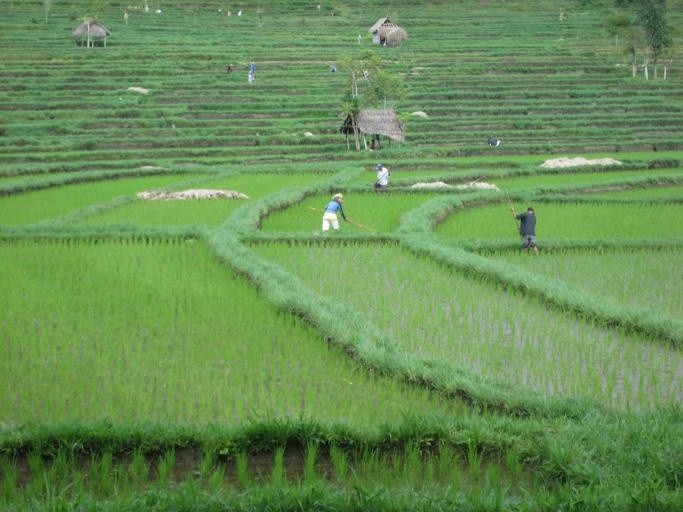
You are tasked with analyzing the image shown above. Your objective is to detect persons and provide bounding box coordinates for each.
[322,193,347,232]
[248,59,255,81]
[510,207,538,254]
[376,164,390,187]
[487,137,501,146]
